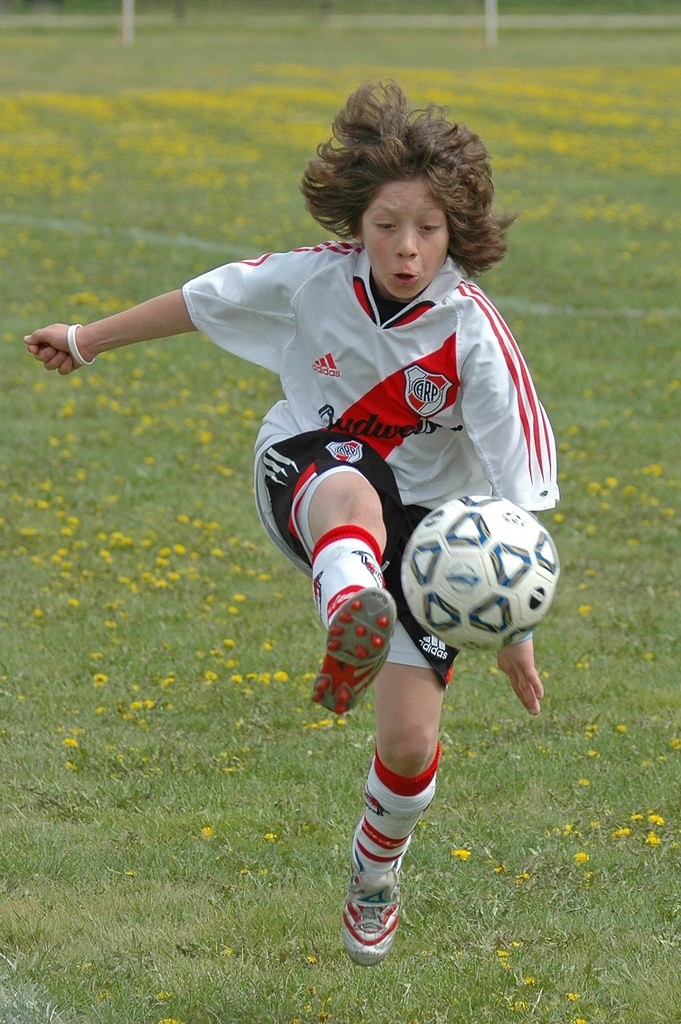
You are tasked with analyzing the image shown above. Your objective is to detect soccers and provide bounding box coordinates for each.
[401,493,562,651]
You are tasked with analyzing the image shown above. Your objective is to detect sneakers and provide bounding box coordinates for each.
[311,587,397,715]
[342,815,403,966]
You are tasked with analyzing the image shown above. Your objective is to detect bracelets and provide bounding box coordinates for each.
[66,324,97,366]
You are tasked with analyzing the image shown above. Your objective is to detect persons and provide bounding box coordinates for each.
[24,80,561,962]
